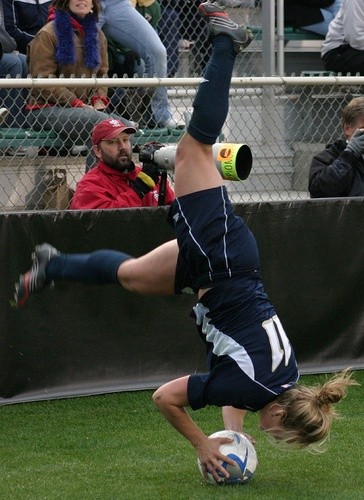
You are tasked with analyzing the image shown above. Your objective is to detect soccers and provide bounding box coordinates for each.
[197,430,257,483]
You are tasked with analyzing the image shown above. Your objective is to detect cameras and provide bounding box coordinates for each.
[133,141,253,182]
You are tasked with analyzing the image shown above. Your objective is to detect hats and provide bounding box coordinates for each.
[92,119,136,144]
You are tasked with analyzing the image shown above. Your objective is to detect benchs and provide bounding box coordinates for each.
[0,18,326,188]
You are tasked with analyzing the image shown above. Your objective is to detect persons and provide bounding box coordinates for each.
[2,0,53,55]
[100,0,161,119]
[0,0,27,127]
[321,0,364,95]
[26,0,135,174]
[95,0,186,129]
[67,118,177,210]
[308,95,364,198]
[283,0,341,36]
[10,0,362,480]
[155,0,215,78]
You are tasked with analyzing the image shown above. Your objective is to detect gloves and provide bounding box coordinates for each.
[135,162,162,196]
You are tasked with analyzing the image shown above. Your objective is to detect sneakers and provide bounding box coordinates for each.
[199,0,254,54]
[9,243,60,307]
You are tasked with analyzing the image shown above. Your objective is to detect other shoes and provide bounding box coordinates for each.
[166,118,185,128]
[5,145,27,155]
[72,145,88,154]
[0,108,9,125]
